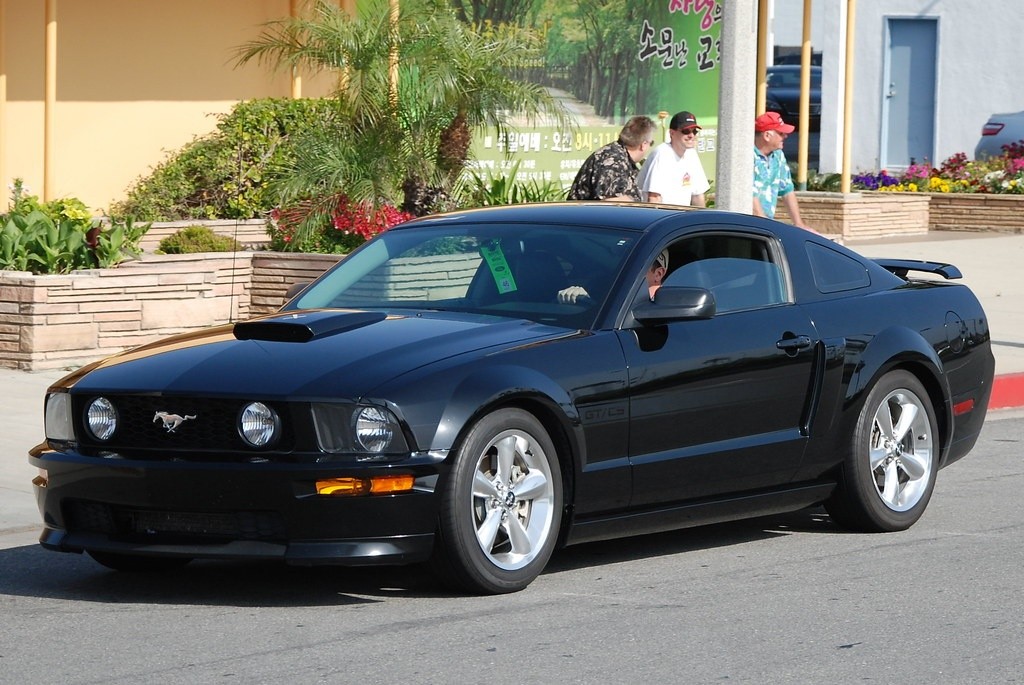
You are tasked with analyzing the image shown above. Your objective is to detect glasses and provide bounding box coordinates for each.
[675,129,697,135]
[646,140,654,147]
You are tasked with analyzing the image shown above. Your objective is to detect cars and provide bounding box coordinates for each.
[767,63,822,132]
[27,200,994,596]
[975,111,1024,164]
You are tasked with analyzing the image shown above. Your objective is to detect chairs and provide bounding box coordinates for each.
[490,250,566,304]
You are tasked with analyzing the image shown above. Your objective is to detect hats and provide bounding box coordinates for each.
[656,248,669,271]
[670,111,702,130]
[755,112,795,134]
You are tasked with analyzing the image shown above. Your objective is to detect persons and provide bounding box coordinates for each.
[752,111,819,233]
[636,111,712,208]
[565,115,658,204]
[557,237,670,303]
